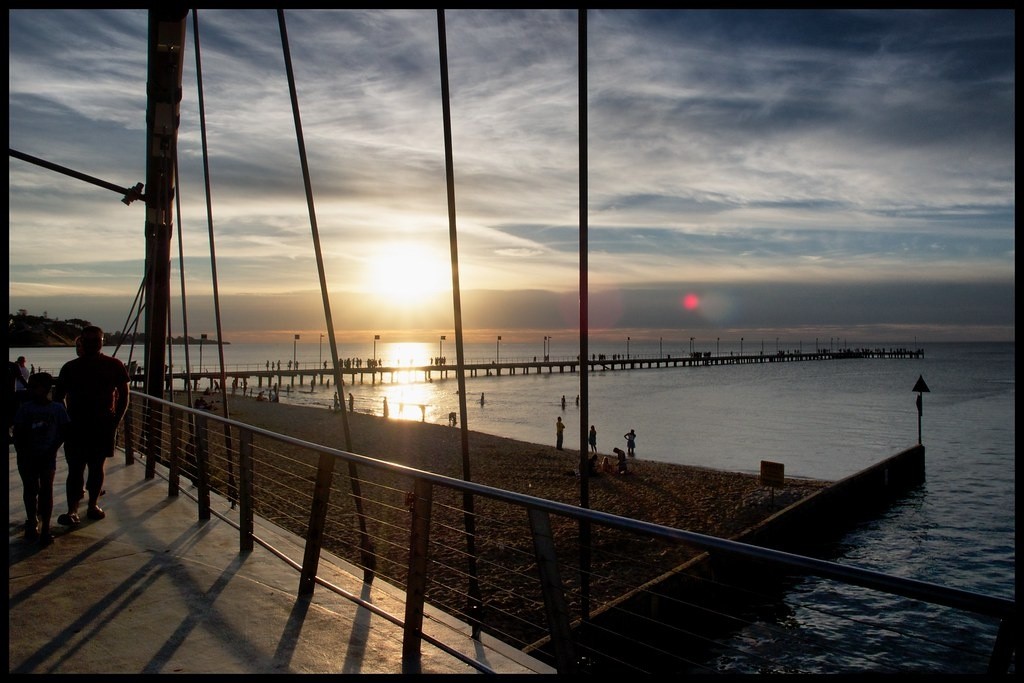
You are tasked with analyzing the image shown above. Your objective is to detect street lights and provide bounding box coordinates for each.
[497,335,503,364]
[543,336,548,355]
[199,333,207,372]
[373,334,381,360]
[293,334,299,363]
[622,334,847,357]
[439,335,446,359]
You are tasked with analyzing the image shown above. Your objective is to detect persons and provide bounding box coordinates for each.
[136,356,459,426]
[52,326,131,525]
[9,356,67,543]
[480,347,925,475]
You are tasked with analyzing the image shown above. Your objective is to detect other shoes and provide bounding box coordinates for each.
[41,528,55,540]
[87,506,105,519]
[57,513,81,525]
[25,520,42,543]
[85,483,106,496]
